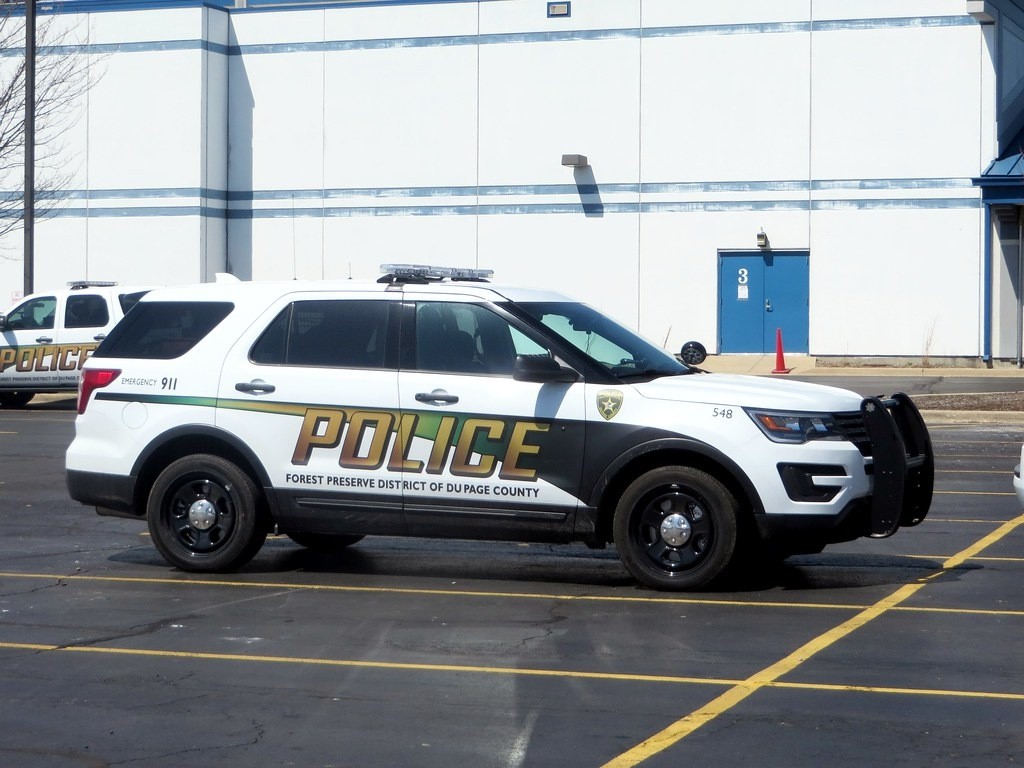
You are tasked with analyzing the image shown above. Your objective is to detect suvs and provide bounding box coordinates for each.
[63,261,936,591]
[0,280,153,413]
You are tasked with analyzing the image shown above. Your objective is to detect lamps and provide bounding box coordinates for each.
[756,232,765,247]
[561,154,588,167]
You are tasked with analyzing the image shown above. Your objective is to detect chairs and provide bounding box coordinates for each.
[438,307,478,356]
[416,306,445,370]
